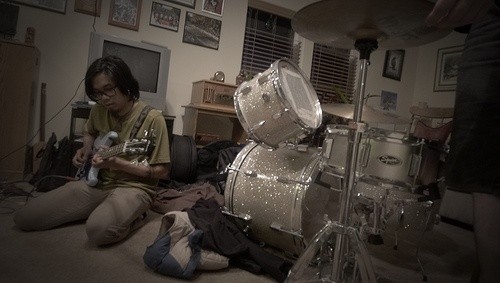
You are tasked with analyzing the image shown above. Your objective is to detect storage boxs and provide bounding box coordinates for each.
[191,79,238,105]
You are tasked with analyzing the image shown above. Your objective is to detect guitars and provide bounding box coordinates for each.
[74,129,156,186]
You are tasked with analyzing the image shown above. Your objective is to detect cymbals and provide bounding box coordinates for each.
[290,1,454,51]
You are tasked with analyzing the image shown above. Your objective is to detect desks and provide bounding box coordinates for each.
[180,104,249,148]
[69,103,176,147]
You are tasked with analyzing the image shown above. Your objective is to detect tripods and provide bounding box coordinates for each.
[284,40,385,283]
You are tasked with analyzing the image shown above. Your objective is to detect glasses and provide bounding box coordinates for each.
[89,85,118,101]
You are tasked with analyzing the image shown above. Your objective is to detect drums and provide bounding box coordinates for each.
[220,140,344,267]
[355,126,447,199]
[233,58,322,151]
[323,123,353,182]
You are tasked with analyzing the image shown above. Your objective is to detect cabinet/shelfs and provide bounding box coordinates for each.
[0,38,42,181]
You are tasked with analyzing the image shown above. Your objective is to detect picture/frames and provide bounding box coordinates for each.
[149,1,181,32]
[109,0,142,32]
[382,49,406,81]
[202,0,224,17]
[4,0,68,15]
[433,44,465,92]
[74,0,102,18]
[182,11,222,51]
[164,0,196,9]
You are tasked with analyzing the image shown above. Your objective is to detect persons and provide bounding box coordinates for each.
[13,55,170,245]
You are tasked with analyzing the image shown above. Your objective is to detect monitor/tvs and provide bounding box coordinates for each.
[88,32,171,114]
